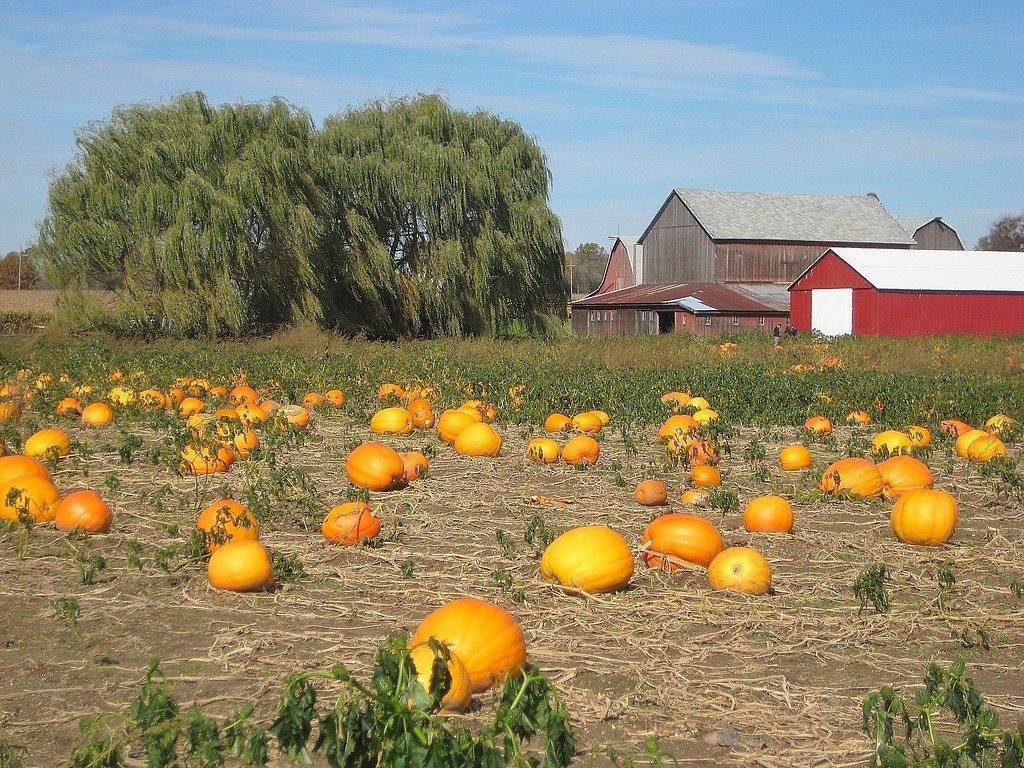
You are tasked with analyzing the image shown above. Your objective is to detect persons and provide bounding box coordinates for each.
[785,324,797,337]
[774,324,781,347]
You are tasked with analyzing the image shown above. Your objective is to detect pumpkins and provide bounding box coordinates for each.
[389,640,472,716]
[0,342,1017,594]
[410,598,527,693]
